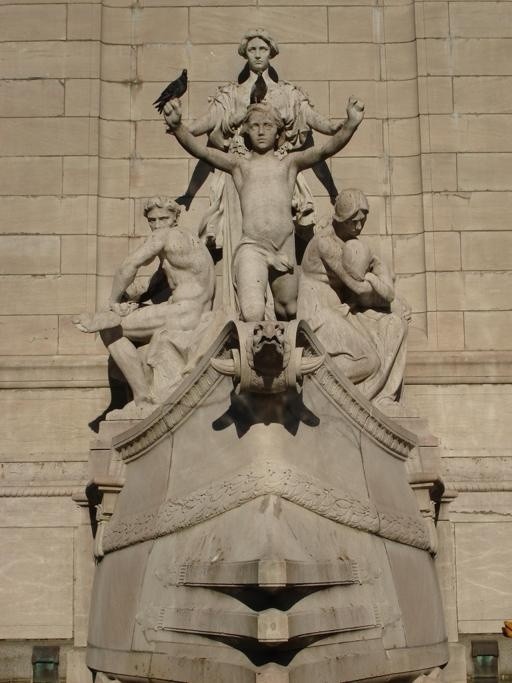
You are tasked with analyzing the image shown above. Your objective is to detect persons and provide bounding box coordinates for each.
[164,92,365,322]
[163,28,349,321]
[73,194,215,408]
[297,185,412,404]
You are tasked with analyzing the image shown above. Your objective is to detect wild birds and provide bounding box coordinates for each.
[152,67,189,116]
